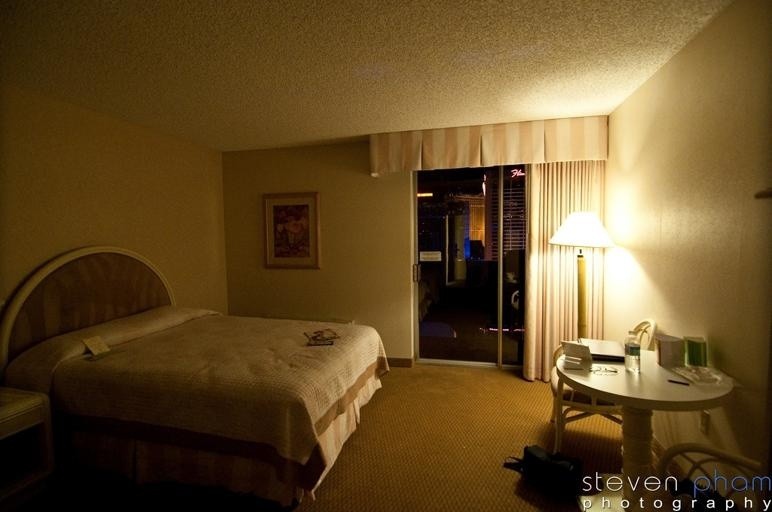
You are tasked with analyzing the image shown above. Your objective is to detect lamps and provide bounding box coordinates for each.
[548,211,613,339]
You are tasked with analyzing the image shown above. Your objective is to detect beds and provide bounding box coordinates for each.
[419,282,432,322]
[0,245,390,512]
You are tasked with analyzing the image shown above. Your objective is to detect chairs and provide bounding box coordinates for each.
[635,442,772,512]
[551,321,651,453]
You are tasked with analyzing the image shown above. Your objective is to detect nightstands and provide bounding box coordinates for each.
[0,388,51,504]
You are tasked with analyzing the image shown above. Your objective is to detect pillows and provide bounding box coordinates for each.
[18,305,200,366]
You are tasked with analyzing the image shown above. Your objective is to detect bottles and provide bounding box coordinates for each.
[625,331,640,376]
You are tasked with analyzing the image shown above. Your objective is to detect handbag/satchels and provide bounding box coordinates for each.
[521,445,586,504]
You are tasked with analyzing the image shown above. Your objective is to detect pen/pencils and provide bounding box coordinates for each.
[667,380,689,385]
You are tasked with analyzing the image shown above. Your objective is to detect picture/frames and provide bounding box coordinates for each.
[262,192,321,271]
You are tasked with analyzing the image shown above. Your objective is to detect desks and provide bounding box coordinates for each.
[556,350,736,512]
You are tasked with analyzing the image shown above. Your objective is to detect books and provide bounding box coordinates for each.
[575,336,626,362]
